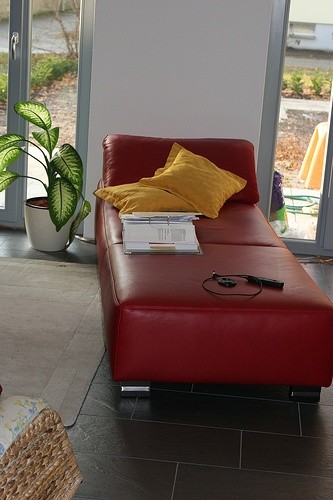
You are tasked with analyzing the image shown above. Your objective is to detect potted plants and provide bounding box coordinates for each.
[0,99,91,252]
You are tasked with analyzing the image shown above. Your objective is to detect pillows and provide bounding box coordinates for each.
[94,143,247,219]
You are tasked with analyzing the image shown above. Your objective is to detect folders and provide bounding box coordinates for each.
[120,211,203,256]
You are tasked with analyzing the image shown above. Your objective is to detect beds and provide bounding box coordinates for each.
[94,135,332,401]
